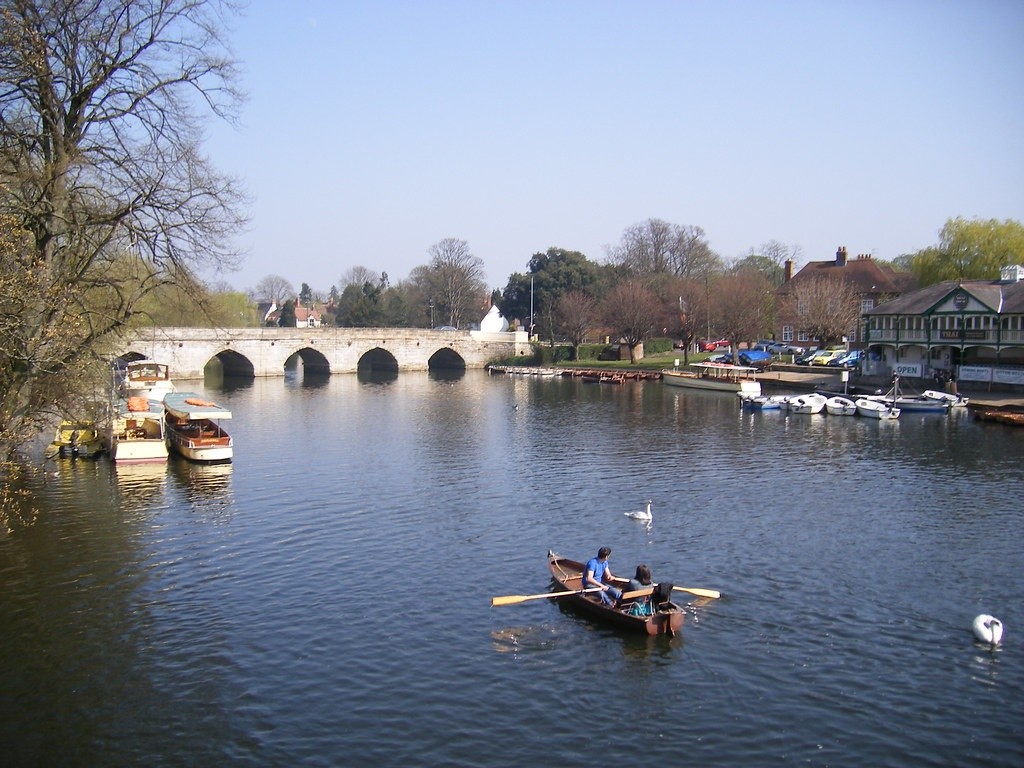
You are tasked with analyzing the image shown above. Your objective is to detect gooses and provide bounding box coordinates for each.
[624,500,653,519]
[632,518,653,531]
[974,614,1003,645]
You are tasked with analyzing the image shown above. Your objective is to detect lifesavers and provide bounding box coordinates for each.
[724,364,731,366]
[185,397,215,407]
[702,362,709,364]
[127,395,149,412]
[711,363,721,366]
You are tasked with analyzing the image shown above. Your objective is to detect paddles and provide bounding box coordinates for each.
[607,576,720,599]
[492,587,609,606]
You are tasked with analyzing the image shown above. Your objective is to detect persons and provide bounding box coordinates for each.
[618,564,653,608]
[934,371,955,383]
[582,547,622,606]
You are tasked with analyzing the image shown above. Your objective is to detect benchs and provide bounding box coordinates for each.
[616,587,654,613]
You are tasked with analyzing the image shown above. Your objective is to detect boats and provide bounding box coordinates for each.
[116,359,178,404]
[546,548,687,634]
[826,395,857,416]
[161,390,234,460]
[788,393,827,415]
[106,397,169,461]
[736,391,781,411]
[922,389,969,407]
[52,418,97,445]
[855,397,901,419]
[661,362,761,397]
[854,371,959,412]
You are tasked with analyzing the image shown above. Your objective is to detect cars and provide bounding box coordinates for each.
[433,325,457,331]
[829,350,865,369]
[754,339,805,355]
[812,350,846,366]
[794,350,825,366]
[673,336,731,353]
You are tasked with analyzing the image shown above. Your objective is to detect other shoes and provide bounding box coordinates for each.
[612,600,619,609]
